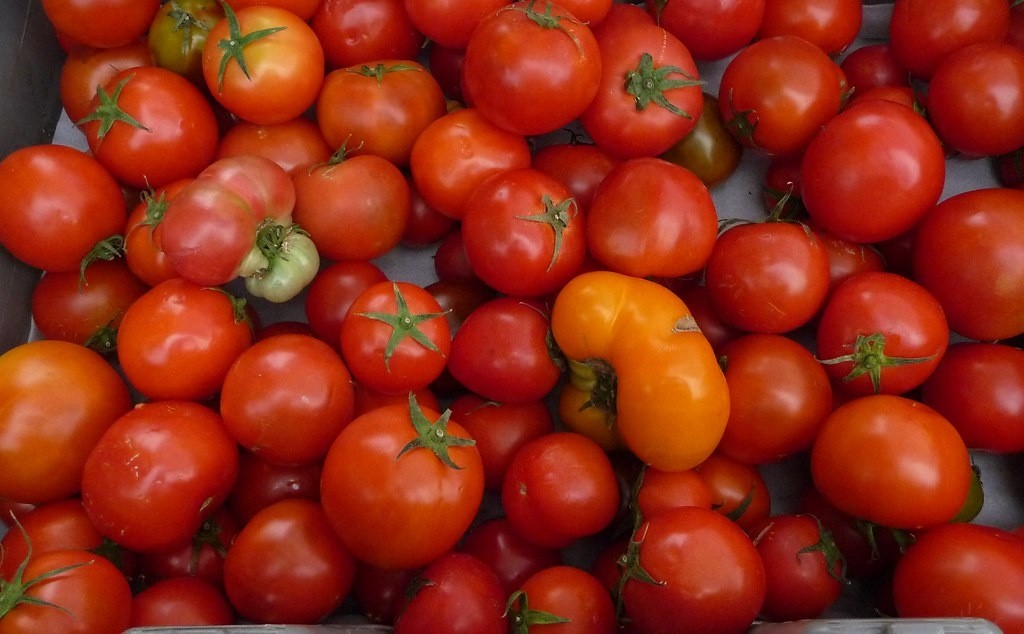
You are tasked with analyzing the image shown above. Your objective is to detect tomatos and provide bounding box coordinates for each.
[0,0,1024,634]
